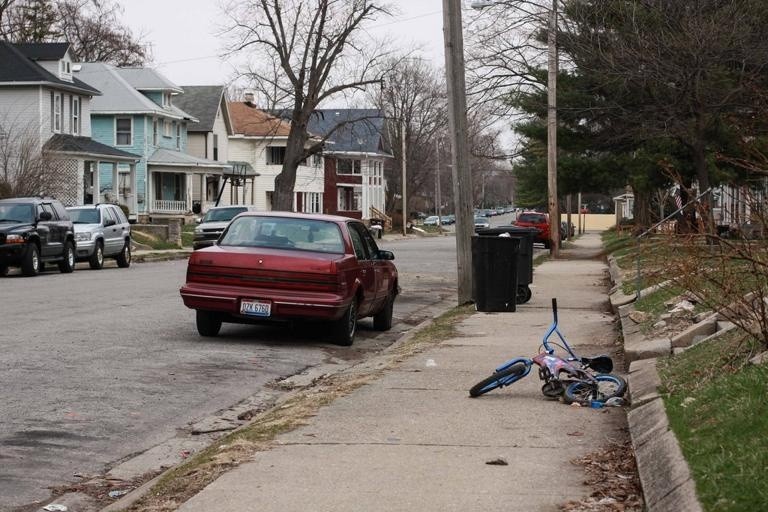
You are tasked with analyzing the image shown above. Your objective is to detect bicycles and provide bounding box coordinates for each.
[470,298,627,406]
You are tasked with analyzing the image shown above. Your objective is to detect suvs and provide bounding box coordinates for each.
[0,196,76,275]
[65,203,131,270]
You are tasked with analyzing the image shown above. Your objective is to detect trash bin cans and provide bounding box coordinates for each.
[471,235,518,313]
[370,217,384,237]
[475,222,545,305]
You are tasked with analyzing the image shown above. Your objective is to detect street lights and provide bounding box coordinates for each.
[472,0,559,260]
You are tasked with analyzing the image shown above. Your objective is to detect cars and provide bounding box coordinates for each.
[193,206,252,250]
[423,214,455,225]
[474,207,519,231]
[180,211,399,345]
[511,212,576,249]
